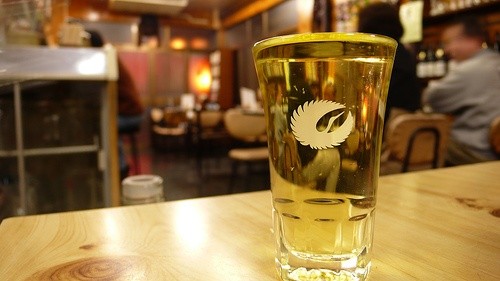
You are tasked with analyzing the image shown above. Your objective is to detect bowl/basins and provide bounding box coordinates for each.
[8,30,42,46]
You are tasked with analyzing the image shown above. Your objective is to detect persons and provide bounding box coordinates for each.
[421,21,500,165]
[272,84,289,176]
[354,4,423,150]
[87,32,144,180]
[288,72,340,192]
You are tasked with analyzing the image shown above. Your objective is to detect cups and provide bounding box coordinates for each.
[252,31,398,281]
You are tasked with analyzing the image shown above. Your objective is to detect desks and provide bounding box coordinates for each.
[0,159,500,281]
[0,44,119,216]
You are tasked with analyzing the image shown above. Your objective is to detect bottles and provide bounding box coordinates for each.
[429,0,488,16]
[59,18,84,45]
[121,174,163,205]
[416,40,456,78]
[481,30,500,50]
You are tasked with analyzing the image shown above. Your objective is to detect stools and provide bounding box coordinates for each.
[117,113,144,178]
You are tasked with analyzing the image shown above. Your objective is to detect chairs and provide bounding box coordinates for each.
[150,106,268,191]
[381,114,453,173]
